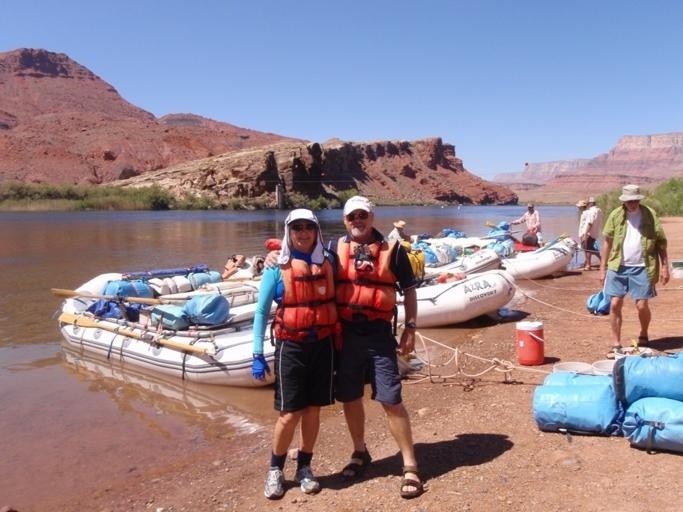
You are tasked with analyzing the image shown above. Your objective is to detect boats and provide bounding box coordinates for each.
[219,253,516,327]
[398,242,506,281]
[433,232,578,279]
[54,263,282,388]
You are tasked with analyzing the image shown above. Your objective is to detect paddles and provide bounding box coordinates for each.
[59,313,204,356]
[51,288,168,304]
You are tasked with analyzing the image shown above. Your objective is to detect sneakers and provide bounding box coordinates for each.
[264,466,285,498]
[294,465,320,493]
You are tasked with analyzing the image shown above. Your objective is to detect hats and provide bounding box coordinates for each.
[576,200,587,207]
[393,221,405,228]
[343,196,373,215]
[619,184,645,202]
[587,197,595,202]
[287,209,317,224]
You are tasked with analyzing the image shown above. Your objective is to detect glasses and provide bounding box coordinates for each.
[346,212,368,221]
[290,225,316,231]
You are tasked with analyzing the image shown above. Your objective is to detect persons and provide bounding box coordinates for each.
[252,209,343,499]
[510,202,543,243]
[264,195,425,500]
[221,254,254,281]
[598,184,669,359]
[576,200,588,268]
[584,197,603,271]
[387,220,413,243]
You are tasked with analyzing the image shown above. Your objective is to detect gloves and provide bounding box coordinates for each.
[252,354,270,379]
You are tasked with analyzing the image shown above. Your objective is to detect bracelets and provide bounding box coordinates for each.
[253,354,263,358]
[405,323,416,329]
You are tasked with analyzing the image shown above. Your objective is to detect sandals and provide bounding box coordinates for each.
[400,465,422,497]
[343,448,372,480]
[607,346,620,359]
[637,336,648,346]
